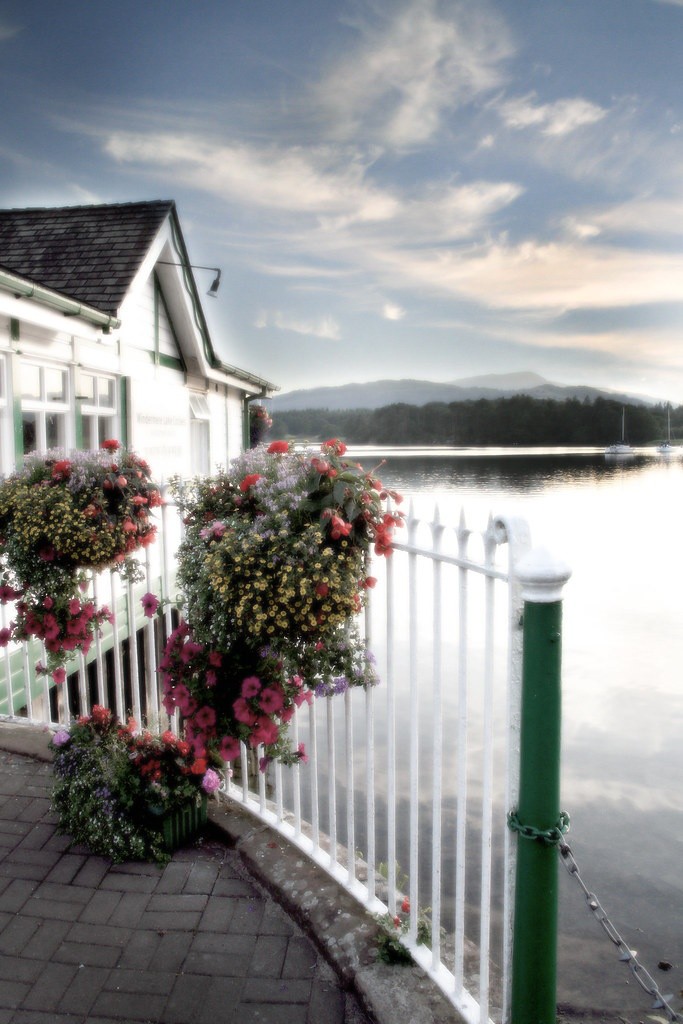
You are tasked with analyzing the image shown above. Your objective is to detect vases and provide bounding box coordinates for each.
[148,793,208,852]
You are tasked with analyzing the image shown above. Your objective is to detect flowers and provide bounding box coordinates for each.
[249,404,273,447]
[0,440,167,683]
[138,435,406,774]
[42,703,235,870]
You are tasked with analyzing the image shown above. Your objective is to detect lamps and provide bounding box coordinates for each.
[157,261,222,299]
